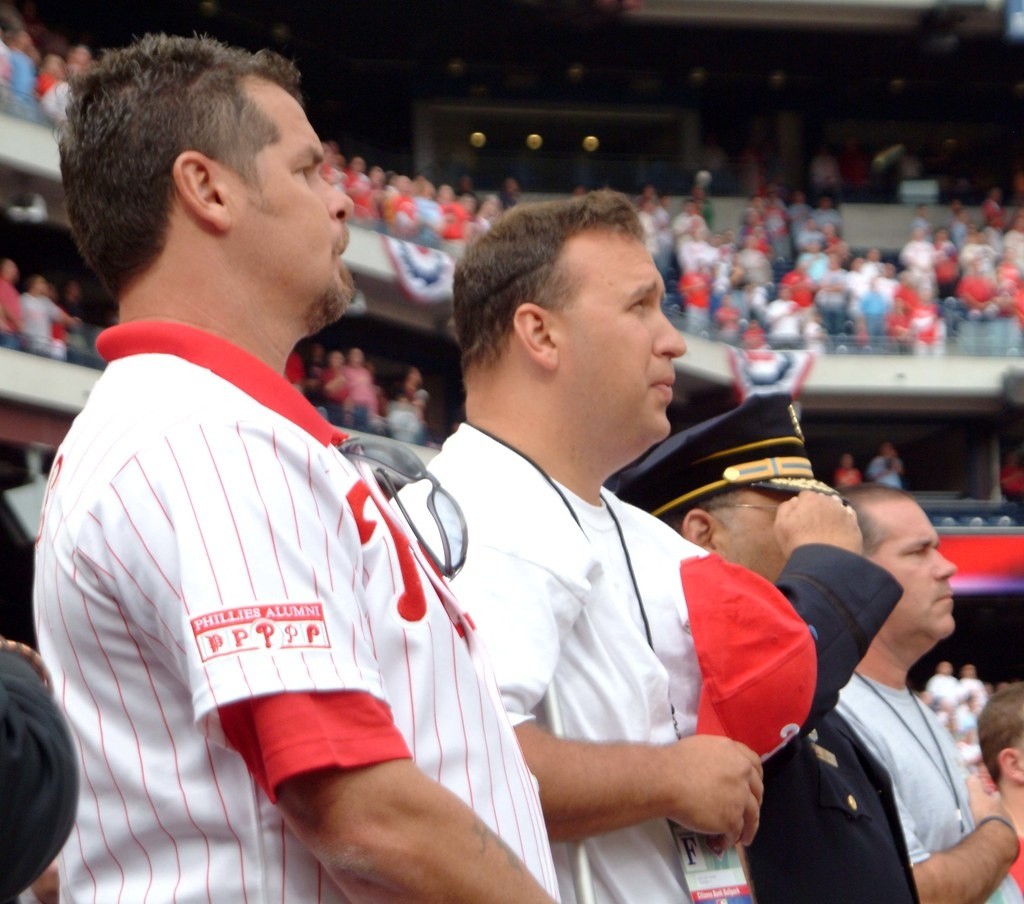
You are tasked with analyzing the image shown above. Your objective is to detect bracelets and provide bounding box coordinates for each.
[977,813,1020,863]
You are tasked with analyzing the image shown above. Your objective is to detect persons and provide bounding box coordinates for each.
[384,192,764,903]
[0,1,1024,903]
[33,33,562,903]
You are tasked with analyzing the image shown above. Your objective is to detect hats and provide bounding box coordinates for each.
[614,393,851,521]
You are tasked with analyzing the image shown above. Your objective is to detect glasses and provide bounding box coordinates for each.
[338,434,468,576]
